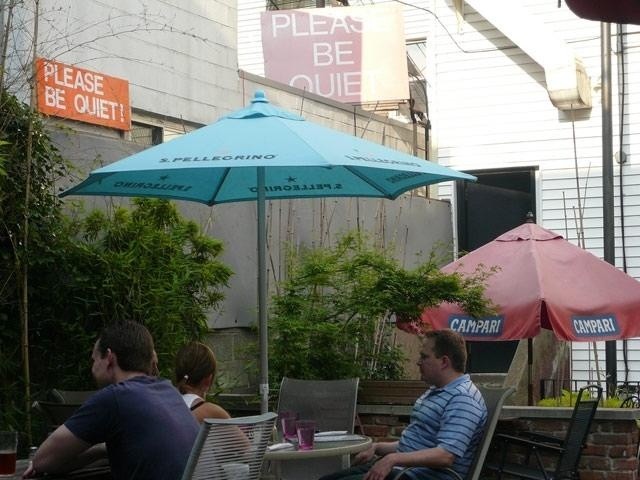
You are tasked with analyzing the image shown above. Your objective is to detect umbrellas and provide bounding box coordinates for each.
[57,88,479,414]
[394,212,640,407]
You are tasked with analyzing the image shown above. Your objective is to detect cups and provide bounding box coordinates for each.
[267,423,278,444]
[279,411,299,442]
[297,420,315,450]
[0,431,18,477]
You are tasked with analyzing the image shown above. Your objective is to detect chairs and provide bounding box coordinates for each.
[275,375,359,433]
[181,411,278,480]
[31,400,81,441]
[367,385,517,480]
[50,389,95,404]
[483,384,602,480]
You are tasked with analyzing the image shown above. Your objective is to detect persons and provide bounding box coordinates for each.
[21,319,223,480]
[174,339,252,479]
[318,327,491,480]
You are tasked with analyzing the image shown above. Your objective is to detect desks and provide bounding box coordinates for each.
[0,456,113,480]
[260,432,373,480]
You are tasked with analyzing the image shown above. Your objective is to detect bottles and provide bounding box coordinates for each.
[28,446,38,466]
